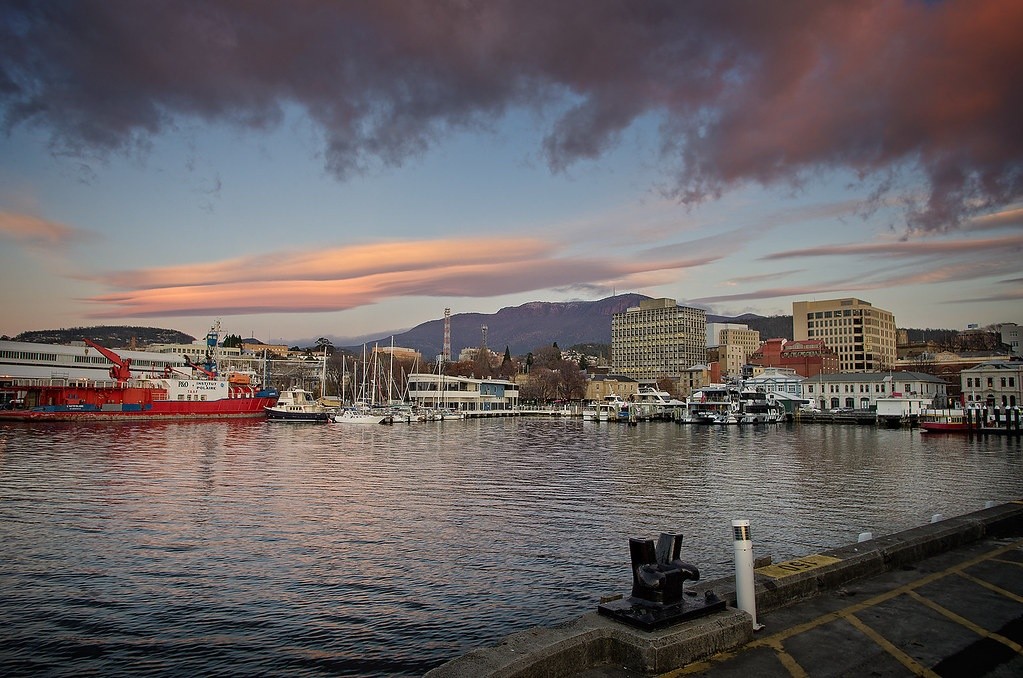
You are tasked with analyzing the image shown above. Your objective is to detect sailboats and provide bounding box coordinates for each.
[333,336,466,423]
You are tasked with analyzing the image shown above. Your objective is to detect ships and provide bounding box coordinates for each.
[0,334,282,422]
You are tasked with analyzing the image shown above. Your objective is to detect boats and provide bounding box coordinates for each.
[918,418,995,430]
[264,387,335,423]
